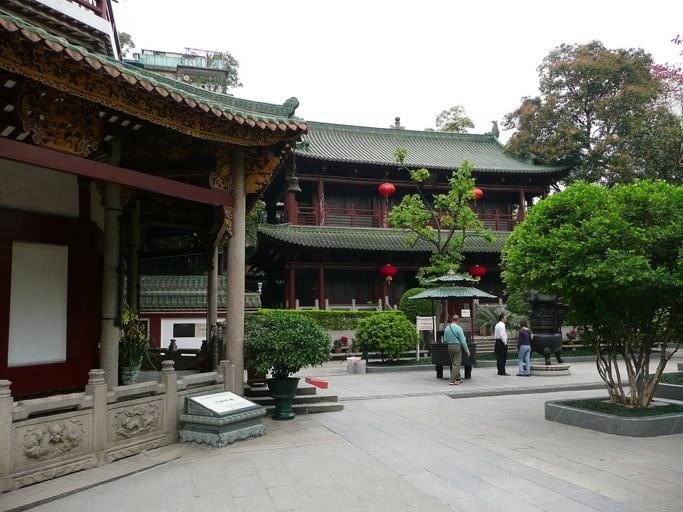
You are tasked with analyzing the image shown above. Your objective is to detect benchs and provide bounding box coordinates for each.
[563,341,668,357]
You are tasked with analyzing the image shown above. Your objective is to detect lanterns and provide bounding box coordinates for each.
[469,264,486,285]
[380,263,397,284]
[470,188,484,208]
[378,182,396,204]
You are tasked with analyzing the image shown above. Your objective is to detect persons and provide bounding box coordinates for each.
[494,314,511,375]
[444,314,470,385]
[517,319,533,376]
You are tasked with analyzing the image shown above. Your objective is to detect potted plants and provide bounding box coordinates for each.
[334,335,360,354]
[119,296,150,386]
[238,305,332,426]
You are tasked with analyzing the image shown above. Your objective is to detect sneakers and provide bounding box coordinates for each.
[457,380,462,383]
[449,382,458,385]
